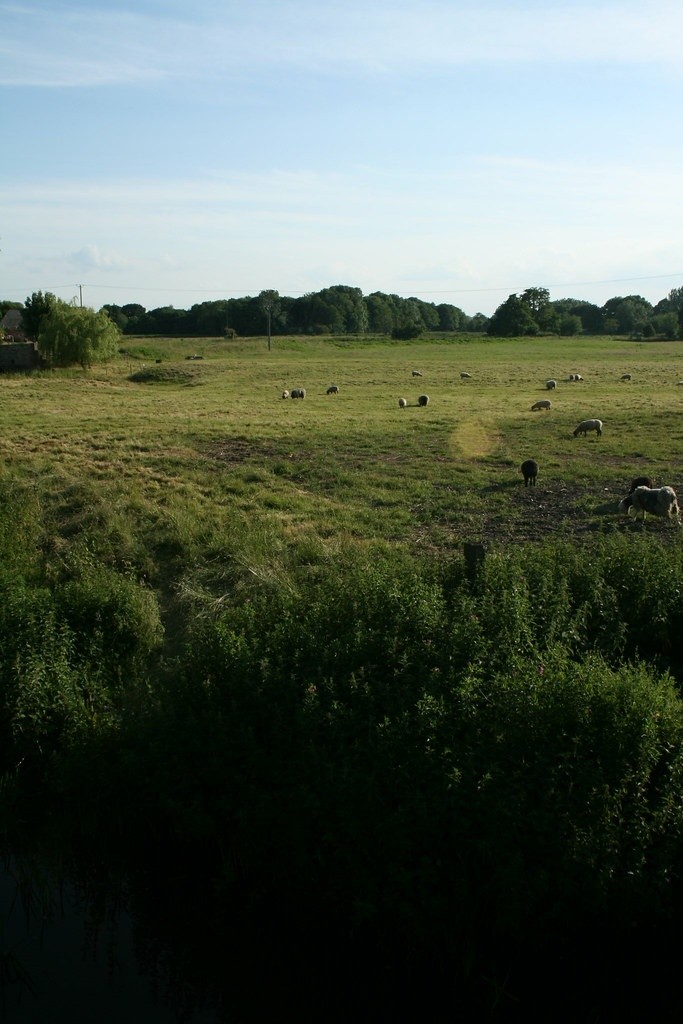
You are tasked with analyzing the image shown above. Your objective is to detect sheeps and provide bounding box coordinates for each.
[282,390,289,399]
[412,371,422,377]
[291,389,306,399]
[547,380,556,390]
[573,419,603,437]
[521,460,538,487]
[620,375,631,381]
[570,374,583,381]
[327,386,339,394]
[459,372,471,379]
[618,477,680,523]
[531,401,551,410]
[399,398,406,407]
[156,354,202,363]
[419,395,428,406]
